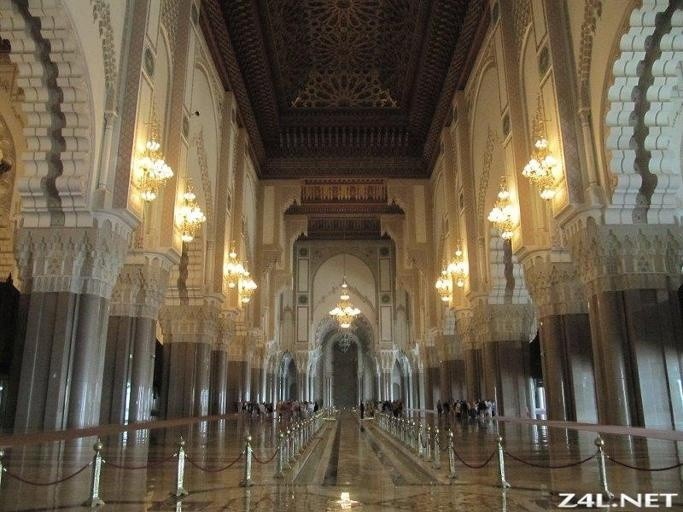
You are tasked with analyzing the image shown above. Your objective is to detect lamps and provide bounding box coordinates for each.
[175,175,206,244]
[521,118,565,201]
[328,226,361,353]
[241,260,257,304]
[138,121,174,204]
[446,240,469,287]
[435,265,453,302]
[486,174,519,240]
[224,239,245,289]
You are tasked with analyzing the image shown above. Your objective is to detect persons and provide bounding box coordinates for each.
[360,399,402,419]
[437,399,496,419]
[232,399,320,421]
